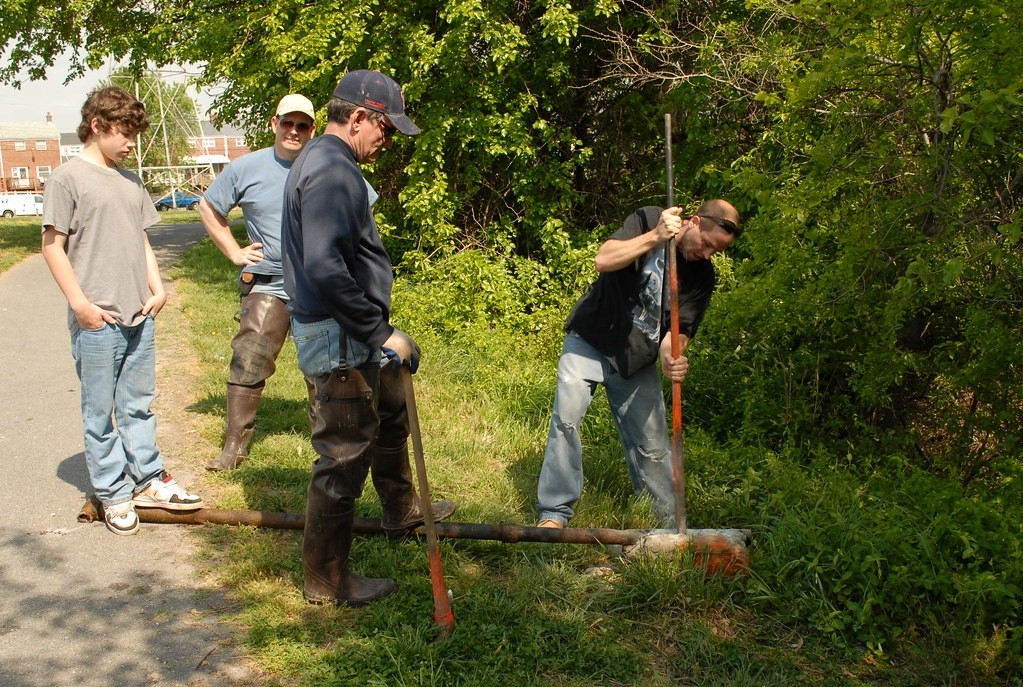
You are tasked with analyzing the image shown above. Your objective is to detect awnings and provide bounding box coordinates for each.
[182,152,232,163]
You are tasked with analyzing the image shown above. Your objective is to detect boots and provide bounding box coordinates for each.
[304,377,318,430]
[372,440,457,530]
[206,385,265,471]
[302,506,395,606]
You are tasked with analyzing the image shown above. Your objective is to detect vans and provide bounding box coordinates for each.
[0,194,44,219]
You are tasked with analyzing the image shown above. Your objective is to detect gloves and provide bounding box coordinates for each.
[380,328,421,375]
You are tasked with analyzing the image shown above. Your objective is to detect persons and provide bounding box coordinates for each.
[41,86,204,537]
[200,91,384,472]
[536,198,742,529]
[279,70,456,606]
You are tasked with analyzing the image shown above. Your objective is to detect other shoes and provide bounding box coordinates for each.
[537,519,564,529]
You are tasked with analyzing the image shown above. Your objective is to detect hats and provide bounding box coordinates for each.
[333,70,421,136]
[275,93,315,121]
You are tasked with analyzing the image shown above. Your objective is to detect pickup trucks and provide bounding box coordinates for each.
[153,191,201,212]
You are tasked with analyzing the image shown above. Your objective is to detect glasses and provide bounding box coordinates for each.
[378,120,397,138]
[699,215,743,238]
[277,118,311,133]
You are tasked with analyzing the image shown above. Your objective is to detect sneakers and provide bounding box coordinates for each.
[103,501,140,535]
[133,471,203,510]
